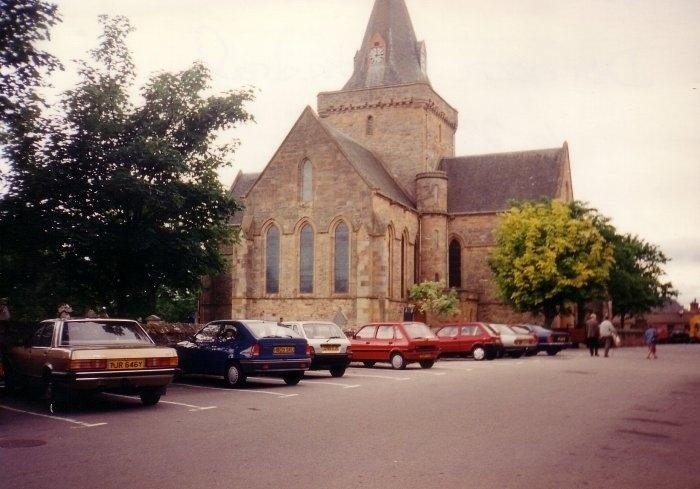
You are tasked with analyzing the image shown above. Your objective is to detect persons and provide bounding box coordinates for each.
[645,324,658,360]
[599,316,618,357]
[584,312,591,331]
[586,314,599,357]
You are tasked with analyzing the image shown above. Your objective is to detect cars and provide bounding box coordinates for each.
[3,314,182,414]
[343,320,445,370]
[273,320,353,380]
[427,321,572,362]
[168,319,314,392]
